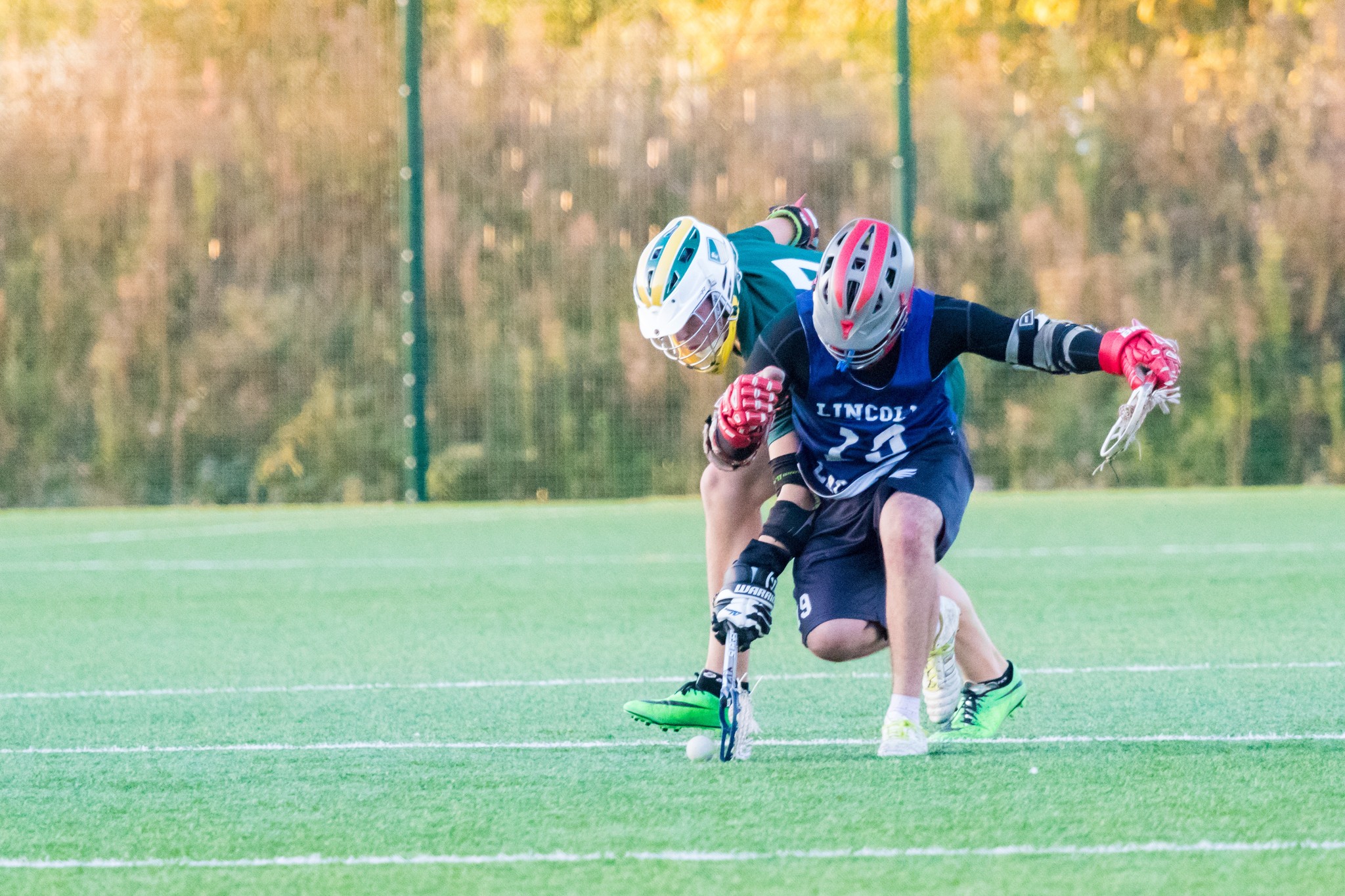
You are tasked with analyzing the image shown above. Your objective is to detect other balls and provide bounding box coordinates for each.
[686,737,714,761]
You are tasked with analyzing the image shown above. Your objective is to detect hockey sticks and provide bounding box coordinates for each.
[719,624,765,764]
[1095,371,1161,471]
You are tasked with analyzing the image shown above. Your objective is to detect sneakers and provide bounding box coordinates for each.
[929,659,1028,741]
[877,715,928,756]
[922,595,963,725]
[622,668,751,733]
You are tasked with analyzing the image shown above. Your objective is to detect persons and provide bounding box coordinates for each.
[702,218,1183,756]
[622,192,1027,748]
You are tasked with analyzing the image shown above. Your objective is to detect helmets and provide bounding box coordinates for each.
[812,219,915,368]
[633,215,740,377]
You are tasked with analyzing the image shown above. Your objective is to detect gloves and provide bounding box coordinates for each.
[766,193,820,250]
[713,561,777,652]
[1099,318,1182,398]
[717,365,785,449]
